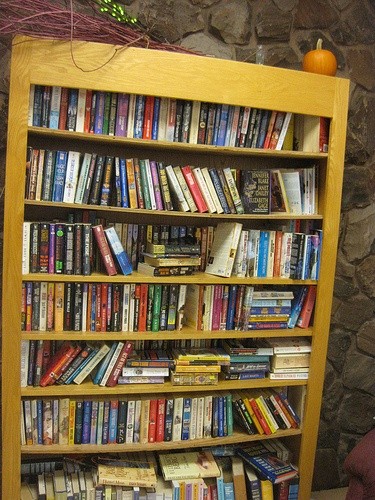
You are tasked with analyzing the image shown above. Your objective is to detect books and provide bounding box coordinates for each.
[21,212,323,281]
[19,339,312,387]
[21,280,317,332]
[28,86,330,154]
[26,145,319,216]
[21,387,303,446]
[21,440,300,500]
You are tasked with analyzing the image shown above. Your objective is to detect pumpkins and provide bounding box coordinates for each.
[302,38,337,77]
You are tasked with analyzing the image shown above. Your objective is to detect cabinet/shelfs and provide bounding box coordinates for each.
[2,33,350,500]
